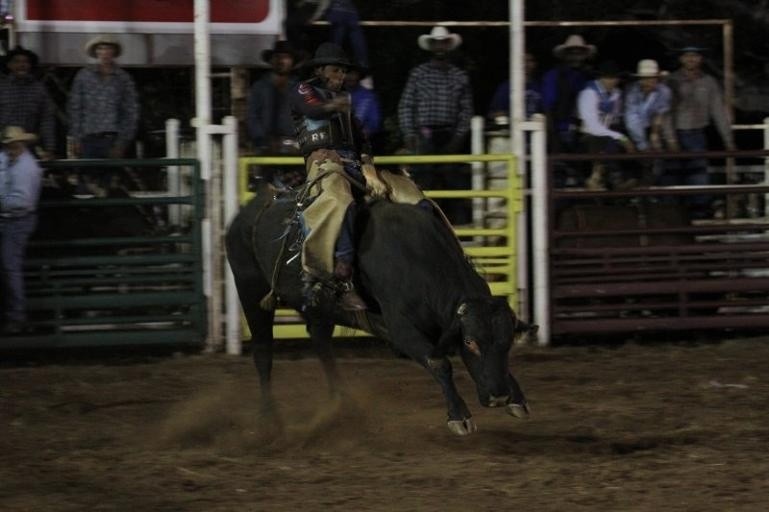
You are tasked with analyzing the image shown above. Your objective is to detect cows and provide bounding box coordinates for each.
[24,186,192,360]
[550,200,725,346]
[225,193,539,436]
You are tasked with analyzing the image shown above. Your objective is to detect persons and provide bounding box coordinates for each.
[340,62,385,155]
[285,40,436,322]
[0,43,58,171]
[66,34,143,194]
[395,23,475,224]
[1,125,46,334]
[247,37,316,199]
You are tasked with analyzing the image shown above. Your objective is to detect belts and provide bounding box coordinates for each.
[92,131,119,137]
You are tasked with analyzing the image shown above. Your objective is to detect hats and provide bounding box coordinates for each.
[630,58,670,78]
[84,34,126,60]
[587,59,628,79]
[2,124,38,146]
[261,39,301,63]
[2,45,40,74]
[306,41,368,80]
[418,24,463,52]
[672,40,707,56]
[552,34,597,60]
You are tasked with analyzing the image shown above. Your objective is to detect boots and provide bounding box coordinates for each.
[335,260,367,312]
